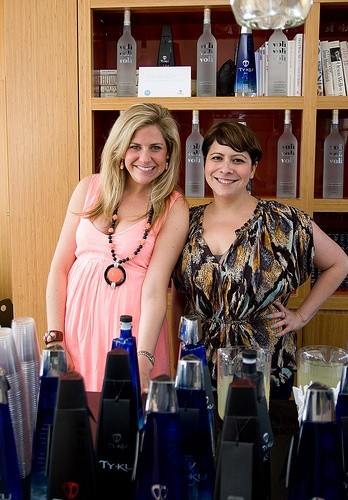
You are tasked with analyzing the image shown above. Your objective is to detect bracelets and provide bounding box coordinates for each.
[138,351,155,365]
[44,330,63,345]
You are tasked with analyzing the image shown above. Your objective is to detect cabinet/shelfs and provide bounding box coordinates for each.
[77,0,348,312]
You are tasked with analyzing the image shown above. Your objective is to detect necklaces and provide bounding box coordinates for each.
[104,202,155,289]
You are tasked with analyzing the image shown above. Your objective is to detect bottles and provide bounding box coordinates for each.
[0,368,29,500]
[276,110,297,199]
[240,114,252,195]
[322,109,343,200]
[233,25,256,97]
[185,110,206,199]
[268,28,290,97]
[31,316,348,500]
[156,24,175,67]
[116,10,137,97]
[197,8,217,97]
[311,233,348,291]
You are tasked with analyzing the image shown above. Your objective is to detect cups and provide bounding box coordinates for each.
[0,316,40,479]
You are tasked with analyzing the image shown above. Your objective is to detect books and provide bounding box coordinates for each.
[309,223,348,289]
[253,33,348,97]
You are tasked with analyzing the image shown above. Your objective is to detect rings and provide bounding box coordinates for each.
[284,311,287,318]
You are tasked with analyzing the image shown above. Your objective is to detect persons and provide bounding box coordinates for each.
[45,102,190,393]
[173,121,348,404]
